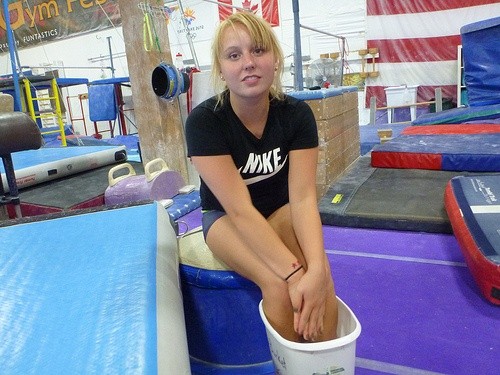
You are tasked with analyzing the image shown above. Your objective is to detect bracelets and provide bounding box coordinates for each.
[285,265,303,281]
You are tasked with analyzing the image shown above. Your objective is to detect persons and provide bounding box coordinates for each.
[185,12,338,343]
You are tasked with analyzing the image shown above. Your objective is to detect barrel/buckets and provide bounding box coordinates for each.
[259,293,362,375]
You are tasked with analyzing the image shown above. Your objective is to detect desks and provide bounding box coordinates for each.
[384,84,420,124]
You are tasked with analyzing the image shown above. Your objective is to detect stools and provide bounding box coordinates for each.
[176,205,277,375]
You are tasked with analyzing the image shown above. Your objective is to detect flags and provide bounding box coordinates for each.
[218,0,279,27]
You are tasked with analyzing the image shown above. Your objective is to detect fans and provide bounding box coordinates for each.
[306,58,339,89]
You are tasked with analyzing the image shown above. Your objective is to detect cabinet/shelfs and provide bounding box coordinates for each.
[457,46,468,108]
